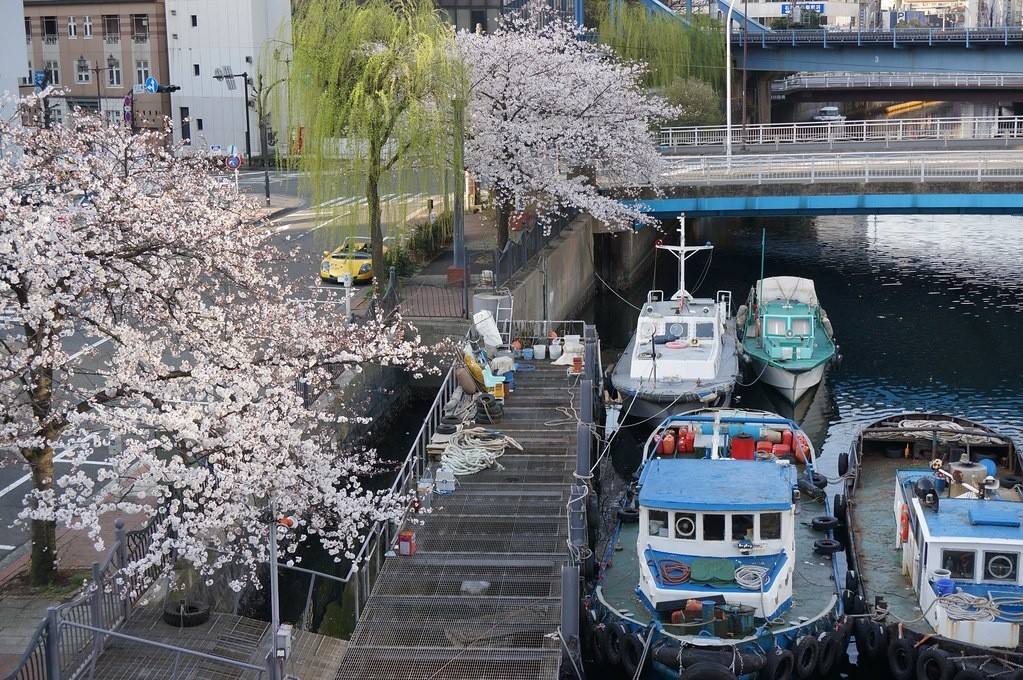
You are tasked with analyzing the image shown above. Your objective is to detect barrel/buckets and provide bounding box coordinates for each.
[932,569,952,582]
[936,579,955,596]
[549,345,562,359]
[533,344,545,360]
[564,335,580,344]
[523,348,533,360]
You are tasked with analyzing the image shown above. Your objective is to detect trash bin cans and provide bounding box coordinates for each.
[715,604,758,638]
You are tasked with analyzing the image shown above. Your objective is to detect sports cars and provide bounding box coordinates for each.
[320,237,389,284]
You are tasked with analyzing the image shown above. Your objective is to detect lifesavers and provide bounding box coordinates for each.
[166,601,209,628]
[476,394,503,424]
[814,540,841,552]
[812,516,837,527]
[437,414,462,434]
[586,600,1023,680]
[999,474,1022,488]
[899,503,909,542]
[837,453,848,476]
[834,494,845,519]
[803,472,827,489]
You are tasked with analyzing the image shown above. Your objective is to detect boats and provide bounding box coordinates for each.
[813,107,847,121]
[835,407,1023,680]
[610,211,744,419]
[742,369,841,460]
[742,228,842,402]
[580,408,854,680]
